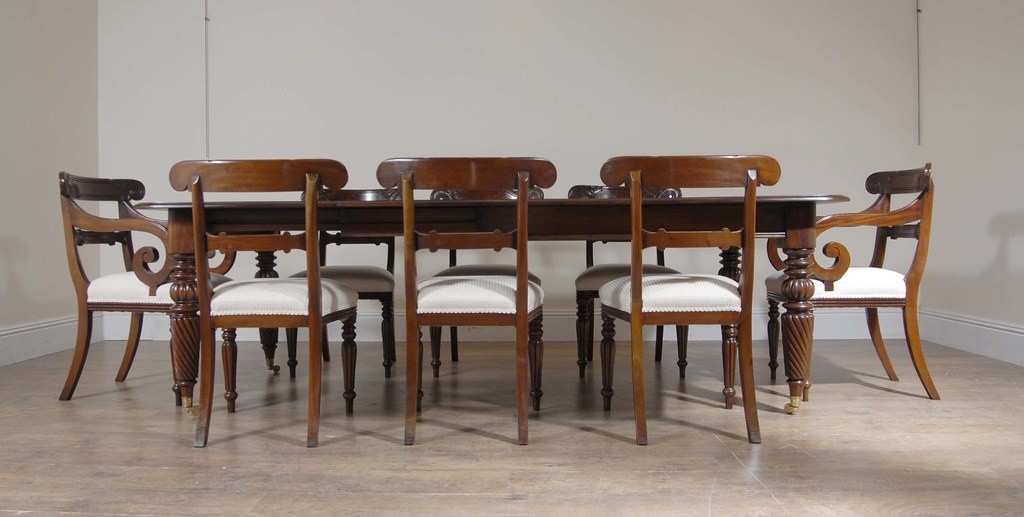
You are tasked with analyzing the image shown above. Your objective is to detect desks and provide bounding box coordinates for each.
[135,196,851,415]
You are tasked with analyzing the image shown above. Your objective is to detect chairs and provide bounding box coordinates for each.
[431,188,544,377]
[766,163,941,402]
[286,189,404,379]
[569,185,689,379]
[169,159,358,447]
[59,171,237,413]
[599,156,780,445]
[376,157,544,445]
